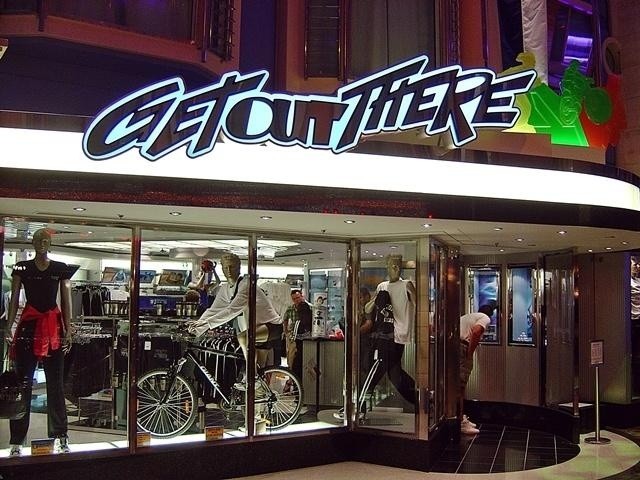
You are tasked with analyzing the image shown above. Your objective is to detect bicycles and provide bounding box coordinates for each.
[136,324,305,439]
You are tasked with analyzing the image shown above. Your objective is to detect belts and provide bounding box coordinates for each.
[460,337,468,347]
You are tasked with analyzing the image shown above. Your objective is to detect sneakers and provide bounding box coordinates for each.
[55,437,71,454]
[9,444,23,458]
[233,378,261,392]
[333,402,367,420]
[460,413,479,434]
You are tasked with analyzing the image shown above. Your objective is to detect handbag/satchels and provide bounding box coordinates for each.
[0,370,29,419]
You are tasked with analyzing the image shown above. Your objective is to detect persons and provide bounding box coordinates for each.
[2,228,72,459]
[166,272,184,286]
[287,289,313,380]
[186,260,221,313]
[455,303,495,437]
[333,257,436,421]
[357,287,378,398]
[184,252,285,394]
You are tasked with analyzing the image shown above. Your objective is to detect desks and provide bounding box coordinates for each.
[78,388,116,429]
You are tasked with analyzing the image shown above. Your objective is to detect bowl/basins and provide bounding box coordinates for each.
[102,299,128,316]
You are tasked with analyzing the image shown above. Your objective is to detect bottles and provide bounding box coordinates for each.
[156,301,163,317]
[173,301,198,317]
[111,371,167,393]
